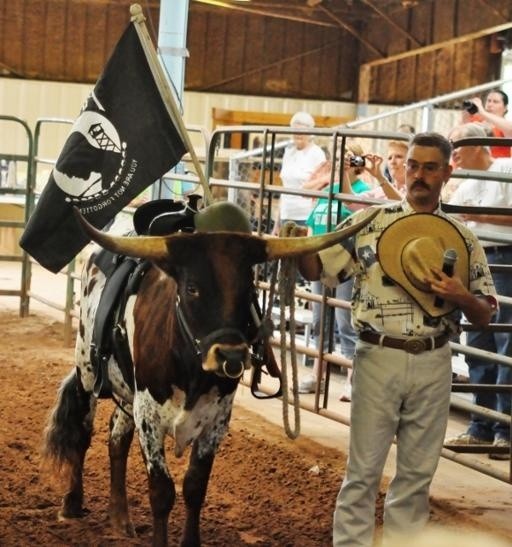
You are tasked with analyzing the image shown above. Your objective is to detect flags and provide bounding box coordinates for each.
[17,22,187,274]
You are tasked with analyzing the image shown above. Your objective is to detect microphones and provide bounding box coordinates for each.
[435,248,457,308]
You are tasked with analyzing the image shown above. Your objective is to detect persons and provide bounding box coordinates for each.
[273,89,512,459]
[297,132,500,547]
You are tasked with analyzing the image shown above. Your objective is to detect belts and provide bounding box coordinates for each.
[358,328,451,354]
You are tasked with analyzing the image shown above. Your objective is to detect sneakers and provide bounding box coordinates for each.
[491,437,512,460]
[290,374,326,393]
[339,383,352,403]
[443,433,492,446]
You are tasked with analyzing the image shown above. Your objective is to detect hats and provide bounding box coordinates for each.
[377,211,470,318]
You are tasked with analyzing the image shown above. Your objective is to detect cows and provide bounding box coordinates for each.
[37,206,382,547]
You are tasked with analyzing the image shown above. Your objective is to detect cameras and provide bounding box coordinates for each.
[349,155,368,168]
[462,97,477,113]
